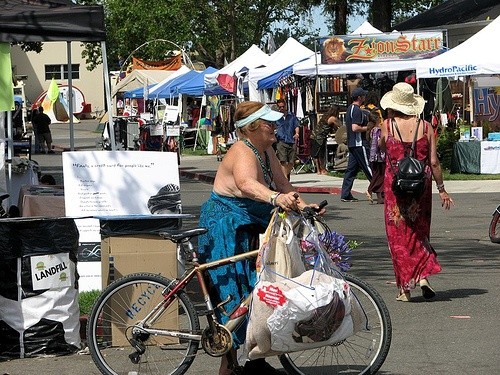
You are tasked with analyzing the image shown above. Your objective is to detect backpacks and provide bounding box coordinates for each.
[385,117,426,199]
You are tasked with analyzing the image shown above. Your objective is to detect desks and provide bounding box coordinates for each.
[451,141,500,175]
[18,184,65,216]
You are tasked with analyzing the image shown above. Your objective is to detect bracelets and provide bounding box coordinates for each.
[437,183,444,190]
[270,192,279,206]
[296,134,299,136]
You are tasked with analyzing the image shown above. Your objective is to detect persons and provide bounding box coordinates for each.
[312,105,343,175]
[342,88,384,202]
[195,101,326,374]
[275,99,301,182]
[365,126,386,203]
[31,107,55,154]
[378,83,455,304]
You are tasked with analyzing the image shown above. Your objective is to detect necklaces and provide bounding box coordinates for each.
[242,138,272,186]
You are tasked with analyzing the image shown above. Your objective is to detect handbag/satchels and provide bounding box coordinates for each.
[255,210,307,283]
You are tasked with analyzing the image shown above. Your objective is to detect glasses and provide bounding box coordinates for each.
[264,121,277,129]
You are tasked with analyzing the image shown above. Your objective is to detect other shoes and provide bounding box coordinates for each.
[397,292,410,302]
[419,279,435,300]
[41,151,46,154]
[320,169,329,174]
[48,150,54,153]
[341,196,359,202]
[244,361,288,375]
[365,190,374,204]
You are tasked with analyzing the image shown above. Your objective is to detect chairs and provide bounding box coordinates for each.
[291,142,315,174]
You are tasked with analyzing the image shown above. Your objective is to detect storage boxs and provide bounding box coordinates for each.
[100,234,181,346]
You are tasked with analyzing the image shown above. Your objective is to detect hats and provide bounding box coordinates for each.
[352,88,369,98]
[233,104,283,128]
[380,82,425,116]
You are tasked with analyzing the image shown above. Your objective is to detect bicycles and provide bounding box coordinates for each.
[85,191,394,375]
[487,203,500,244]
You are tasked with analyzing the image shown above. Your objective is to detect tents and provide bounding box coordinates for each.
[113,22,383,126]
[417,15,500,95]
